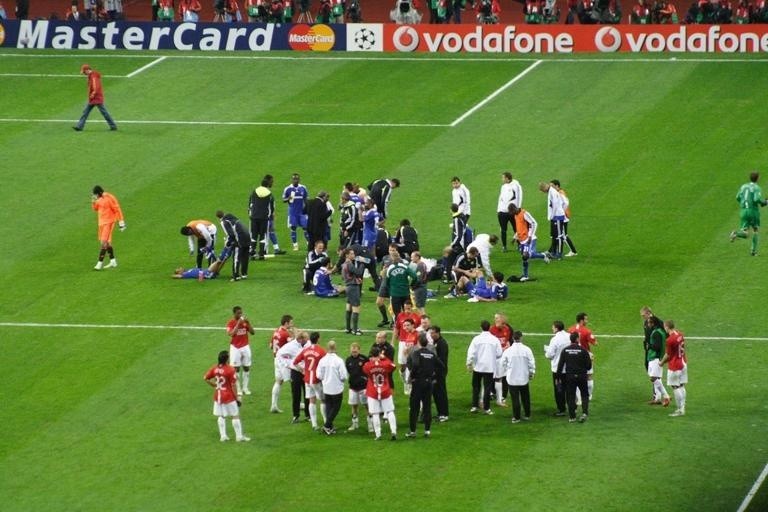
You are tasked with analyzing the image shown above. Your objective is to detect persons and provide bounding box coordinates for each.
[203,350,251,442]
[269,300,599,441]
[640,307,691,418]
[169,170,577,335]
[72,65,118,131]
[90,185,125,270]
[227,304,255,396]
[0,0,768,25]
[729,173,768,257]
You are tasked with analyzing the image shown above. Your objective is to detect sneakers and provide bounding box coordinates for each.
[404,384,409,395]
[501,248,507,252]
[236,390,252,396]
[250,247,299,260]
[648,397,685,417]
[220,437,229,441]
[235,437,252,442]
[555,412,587,422]
[103,263,117,268]
[519,277,530,282]
[94,262,103,270]
[470,393,530,424]
[443,292,455,298]
[271,408,282,413]
[369,286,377,291]
[292,404,449,441]
[378,321,390,327]
[541,249,578,264]
[345,328,362,335]
[229,273,248,281]
